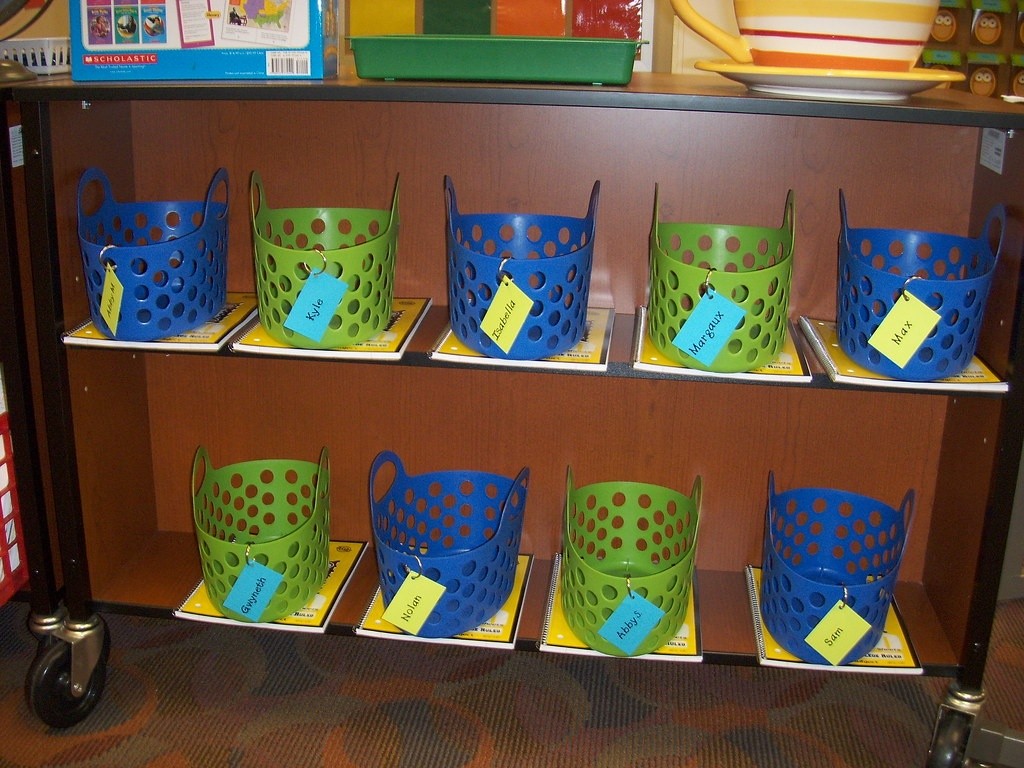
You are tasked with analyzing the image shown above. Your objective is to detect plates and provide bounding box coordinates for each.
[694,58,967,104]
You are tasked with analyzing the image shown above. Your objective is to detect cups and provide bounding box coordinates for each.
[670,0,939,73]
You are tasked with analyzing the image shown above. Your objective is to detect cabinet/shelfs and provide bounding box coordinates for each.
[0,72,1024,768]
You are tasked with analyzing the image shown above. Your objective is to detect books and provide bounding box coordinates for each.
[796,315,1010,394]
[538,551,704,662]
[171,539,369,635]
[630,304,813,384]
[426,305,616,372]
[58,292,259,352]
[228,296,434,359]
[351,552,535,651]
[743,565,924,675]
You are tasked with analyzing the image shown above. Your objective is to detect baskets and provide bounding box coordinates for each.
[77,166,230,342]
[759,471,916,666]
[648,182,795,373]
[369,450,530,638]
[443,174,601,361]
[251,170,401,350]
[560,465,703,657]
[835,188,1006,382]
[191,445,331,623]
